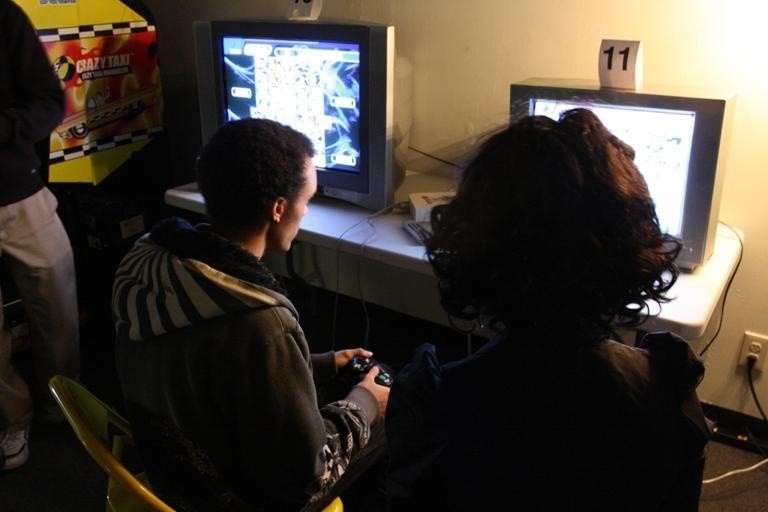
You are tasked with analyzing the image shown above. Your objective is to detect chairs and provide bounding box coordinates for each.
[50,373,344,512]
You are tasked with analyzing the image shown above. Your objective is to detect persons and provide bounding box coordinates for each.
[383,108,715,512]
[108,116,392,510]
[0,0,85,476]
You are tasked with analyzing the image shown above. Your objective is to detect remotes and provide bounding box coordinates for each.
[402,220,433,245]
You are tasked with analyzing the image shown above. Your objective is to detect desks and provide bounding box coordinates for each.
[163,168,744,350]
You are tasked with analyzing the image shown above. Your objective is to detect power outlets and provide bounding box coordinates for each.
[738,332,768,373]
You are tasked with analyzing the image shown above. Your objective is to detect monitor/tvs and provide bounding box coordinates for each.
[508,75,737,272]
[191,18,415,215]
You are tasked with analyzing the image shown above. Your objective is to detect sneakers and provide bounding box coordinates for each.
[0,419,34,471]
